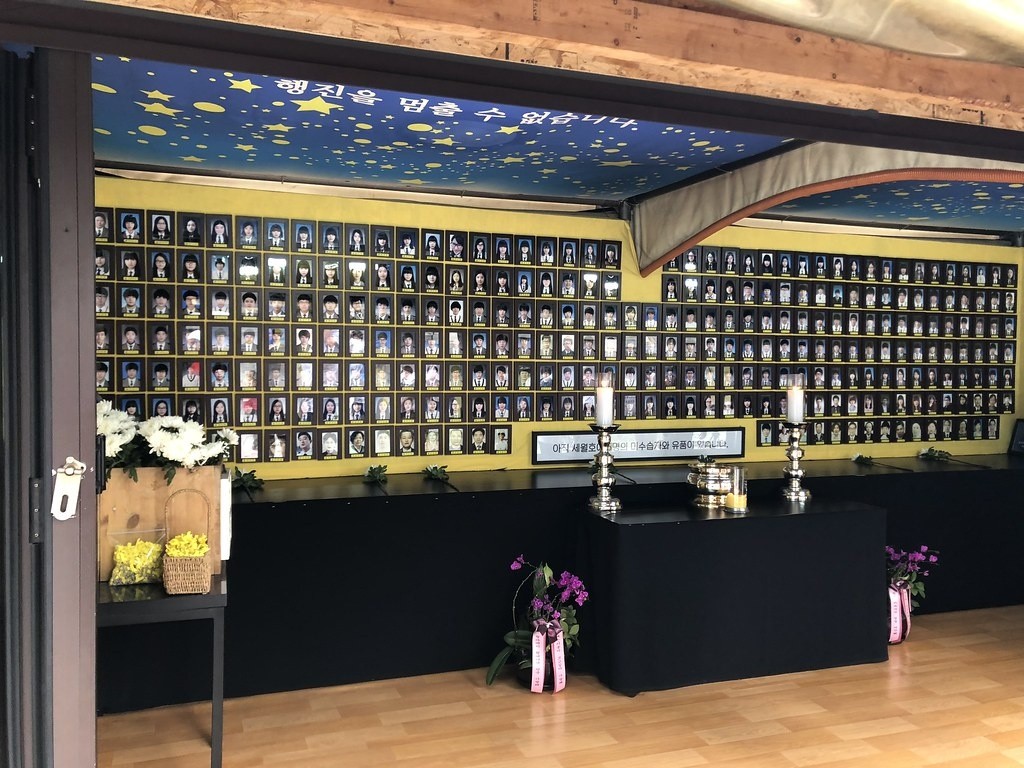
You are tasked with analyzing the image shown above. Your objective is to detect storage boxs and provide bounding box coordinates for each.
[95,469,232,581]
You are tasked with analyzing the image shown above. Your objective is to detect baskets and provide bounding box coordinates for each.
[161,488,213,596]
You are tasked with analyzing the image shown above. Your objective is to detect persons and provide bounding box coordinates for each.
[95,213,1015,459]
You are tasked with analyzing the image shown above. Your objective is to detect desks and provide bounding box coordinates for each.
[95,454,1024,715]
[95,560,227,768]
[587,497,888,697]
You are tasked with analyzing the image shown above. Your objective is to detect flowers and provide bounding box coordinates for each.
[232,466,264,502]
[851,452,913,472]
[486,554,588,694]
[422,465,459,492]
[589,461,637,484]
[918,446,991,469]
[884,544,939,643]
[366,464,387,495]
[96,399,240,486]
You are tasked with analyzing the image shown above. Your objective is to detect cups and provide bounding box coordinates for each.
[725,466,747,513]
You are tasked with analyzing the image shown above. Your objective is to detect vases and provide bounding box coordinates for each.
[517,653,567,691]
[888,613,911,645]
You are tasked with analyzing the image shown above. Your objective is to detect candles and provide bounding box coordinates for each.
[788,386,803,421]
[597,379,613,427]
